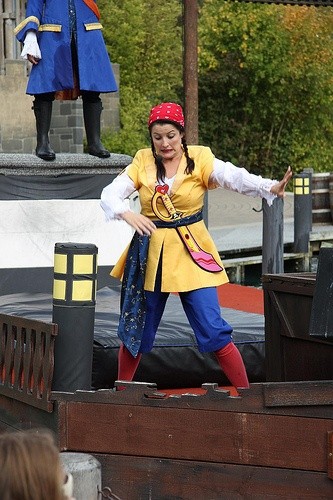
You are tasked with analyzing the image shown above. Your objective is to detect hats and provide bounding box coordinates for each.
[148,103,185,128]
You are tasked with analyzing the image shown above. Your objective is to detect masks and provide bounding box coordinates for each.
[62,472,73,500]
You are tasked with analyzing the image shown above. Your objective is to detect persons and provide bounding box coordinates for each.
[0,431,77,500]
[100,102,294,391]
[12,0,118,160]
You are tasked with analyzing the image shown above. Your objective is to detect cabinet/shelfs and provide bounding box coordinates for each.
[262,272,333,383]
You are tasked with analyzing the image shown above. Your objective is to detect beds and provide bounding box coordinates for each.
[0,152,265,390]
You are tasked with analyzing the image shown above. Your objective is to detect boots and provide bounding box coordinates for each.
[83,102,110,158]
[215,342,250,398]
[31,101,56,160]
[118,342,142,392]
